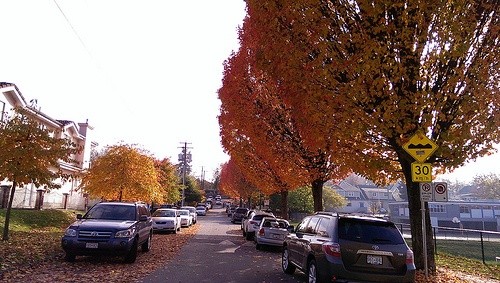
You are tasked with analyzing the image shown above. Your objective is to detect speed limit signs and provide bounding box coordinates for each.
[411,162,433,183]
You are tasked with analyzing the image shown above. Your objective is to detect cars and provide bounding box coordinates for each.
[255,217,291,251]
[196,195,213,216]
[176,210,193,228]
[223,201,249,223]
[151,208,182,234]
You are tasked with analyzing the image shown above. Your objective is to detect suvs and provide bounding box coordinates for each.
[61,199,153,263]
[215,195,222,205]
[281,210,417,283]
[241,209,277,240]
[180,207,198,224]
